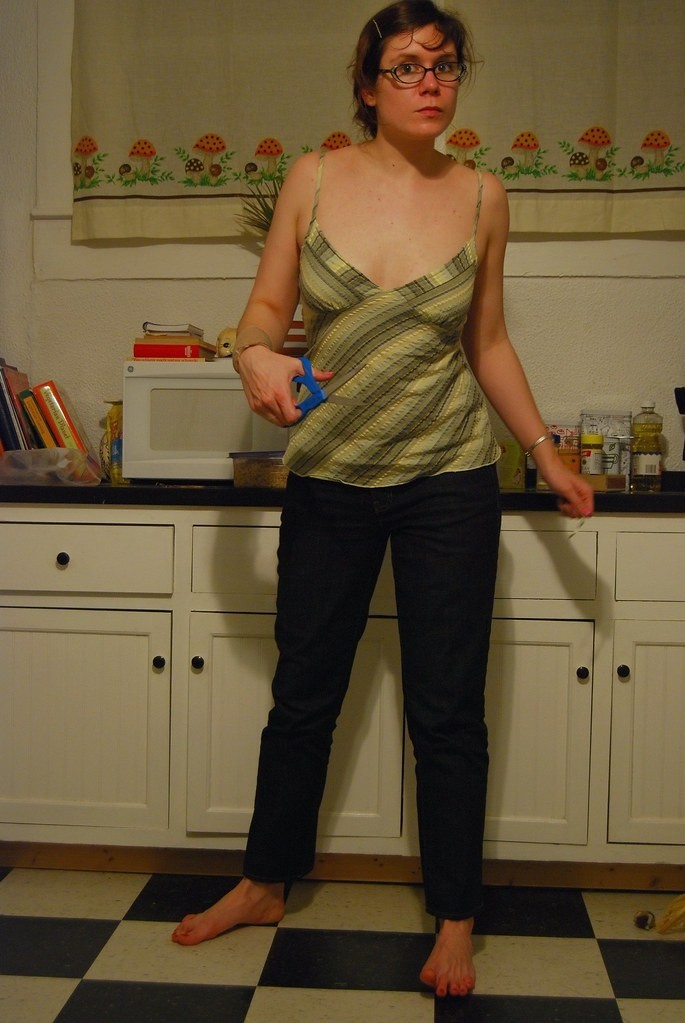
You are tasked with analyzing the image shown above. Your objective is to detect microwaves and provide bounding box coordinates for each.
[121,360,306,481]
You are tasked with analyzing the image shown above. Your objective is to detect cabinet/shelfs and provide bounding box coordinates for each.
[406,512,685,869]
[0,502,406,858]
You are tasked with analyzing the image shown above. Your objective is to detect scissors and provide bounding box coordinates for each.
[282,356,368,429]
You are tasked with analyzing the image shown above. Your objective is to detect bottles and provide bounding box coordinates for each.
[631,401,664,493]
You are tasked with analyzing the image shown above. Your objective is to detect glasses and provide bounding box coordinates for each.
[374,60,466,84]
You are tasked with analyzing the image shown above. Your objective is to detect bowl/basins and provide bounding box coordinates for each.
[226,448,289,490]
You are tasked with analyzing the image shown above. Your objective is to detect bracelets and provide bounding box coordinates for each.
[234,341,272,373]
[525,434,549,457]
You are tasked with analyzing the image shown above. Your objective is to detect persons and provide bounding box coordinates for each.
[170,0,594,996]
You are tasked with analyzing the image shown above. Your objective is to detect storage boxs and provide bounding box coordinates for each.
[228,451,290,488]
[524,410,631,492]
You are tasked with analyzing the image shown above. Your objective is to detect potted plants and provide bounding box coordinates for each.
[234,166,310,356]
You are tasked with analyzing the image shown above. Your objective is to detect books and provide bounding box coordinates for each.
[128,321,218,362]
[0,357,101,484]
